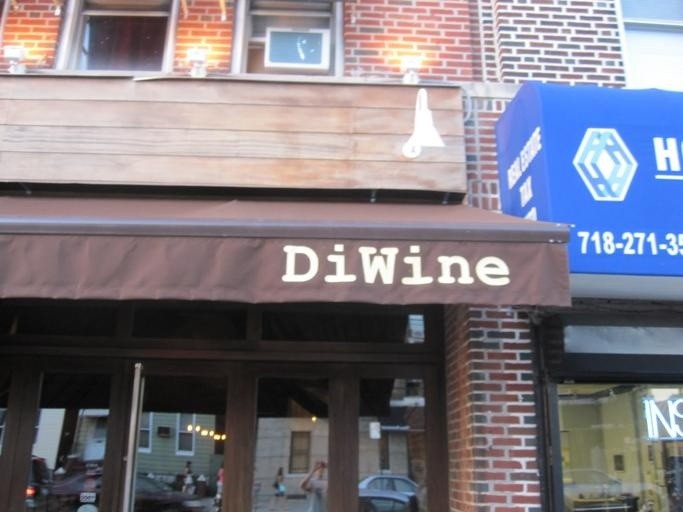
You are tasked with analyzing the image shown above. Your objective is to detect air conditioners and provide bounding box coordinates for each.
[262,26,333,72]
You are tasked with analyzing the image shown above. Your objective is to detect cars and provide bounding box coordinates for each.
[562,468,639,511]
[358,470,420,494]
[53,470,210,512]
[357,489,415,512]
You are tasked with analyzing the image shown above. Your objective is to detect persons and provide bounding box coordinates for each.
[181,460,329,512]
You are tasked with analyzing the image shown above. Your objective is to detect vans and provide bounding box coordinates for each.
[25,456,50,511]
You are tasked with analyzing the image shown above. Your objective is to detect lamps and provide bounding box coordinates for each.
[401,88,447,159]
[401,54,422,85]
[187,47,209,79]
[2,45,28,77]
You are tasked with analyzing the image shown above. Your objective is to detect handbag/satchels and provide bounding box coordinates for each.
[272,480,279,491]
